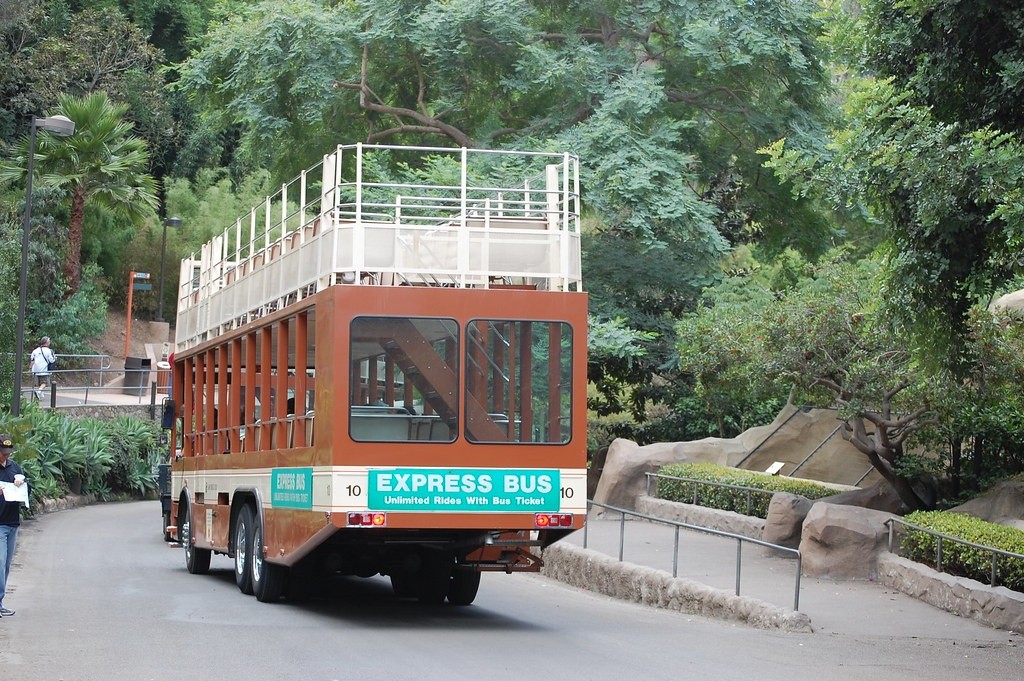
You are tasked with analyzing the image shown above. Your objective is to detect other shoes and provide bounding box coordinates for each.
[35,391,41,400]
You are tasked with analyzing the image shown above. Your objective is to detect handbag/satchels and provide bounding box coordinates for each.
[48,361,58,370]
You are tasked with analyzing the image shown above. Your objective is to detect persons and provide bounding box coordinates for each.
[0,433,31,618]
[30,336,56,400]
[196,408,218,434]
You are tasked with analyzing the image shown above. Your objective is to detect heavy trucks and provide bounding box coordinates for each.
[159,142,589,605]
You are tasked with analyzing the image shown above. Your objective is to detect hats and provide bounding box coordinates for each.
[0,434,14,454]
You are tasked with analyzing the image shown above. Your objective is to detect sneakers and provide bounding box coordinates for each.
[0,606,15,618]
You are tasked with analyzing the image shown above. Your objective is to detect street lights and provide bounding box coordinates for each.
[9,114,76,417]
[156,215,182,323]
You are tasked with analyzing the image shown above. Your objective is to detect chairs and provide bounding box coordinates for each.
[188,215,513,346]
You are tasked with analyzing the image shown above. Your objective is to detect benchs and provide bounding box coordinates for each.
[180,402,509,453]
